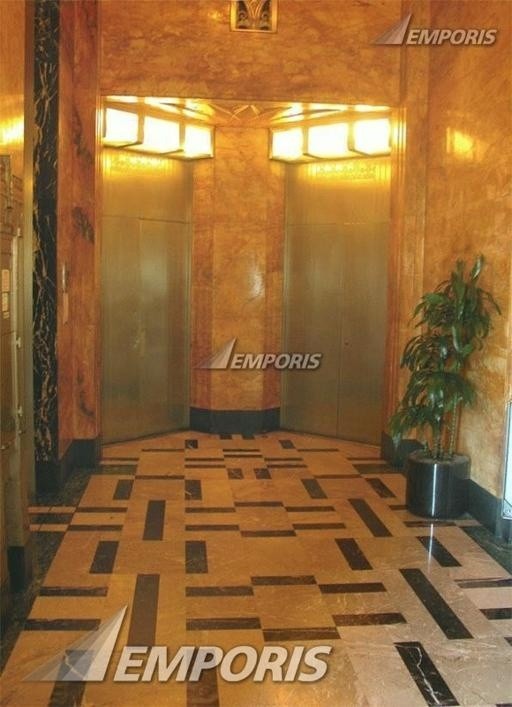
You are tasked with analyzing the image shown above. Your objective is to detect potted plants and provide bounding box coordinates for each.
[390,251,503,519]
